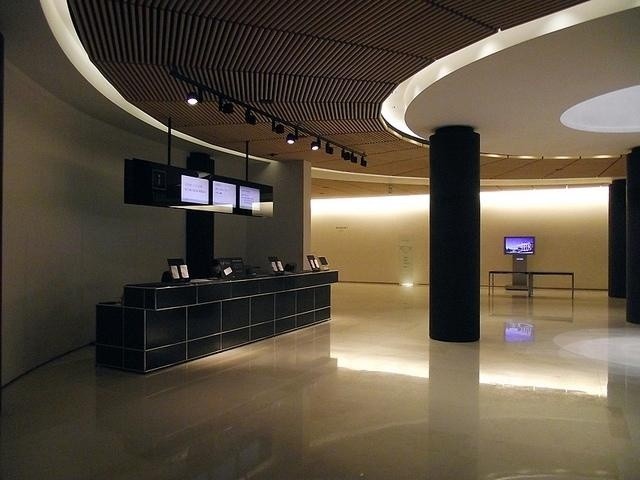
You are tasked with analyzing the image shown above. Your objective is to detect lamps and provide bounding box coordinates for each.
[187,92,367,167]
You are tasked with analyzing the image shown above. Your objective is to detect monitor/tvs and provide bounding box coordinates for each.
[212,180,237,209]
[504,236,536,256]
[503,320,535,342]
[179,174,209,205]
[239,186,260,211]
